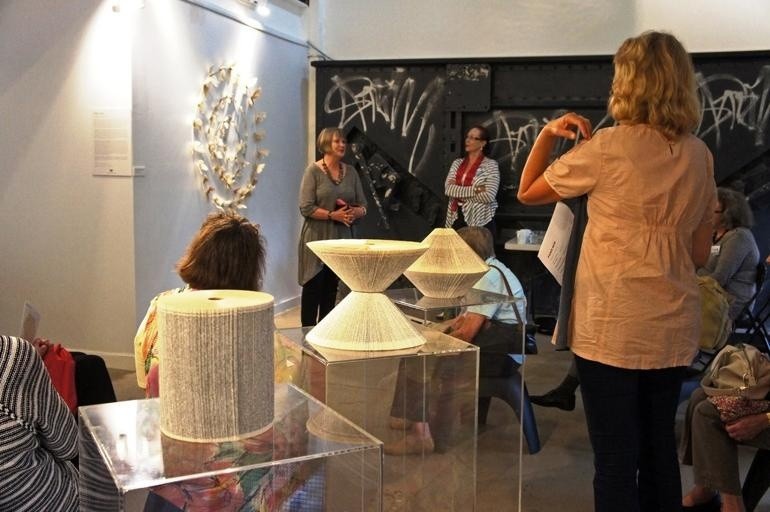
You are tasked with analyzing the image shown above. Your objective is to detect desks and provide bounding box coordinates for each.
[505,236,561,325]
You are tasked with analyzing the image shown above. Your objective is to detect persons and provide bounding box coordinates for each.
[682,254,770,512]
[516,33,717,512]
[383,226,528,455]
[0,335,79,512]
[436,125,501,319]
[297,126,369,358]
[696,187,759,320]
[134,212,303,400]
[530,273,713,411]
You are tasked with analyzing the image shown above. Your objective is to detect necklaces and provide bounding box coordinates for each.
[323,158,344,186]
[712,229,729,245]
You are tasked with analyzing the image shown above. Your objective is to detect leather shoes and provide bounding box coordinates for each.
[383,435,434,455]
[531,390,575,412]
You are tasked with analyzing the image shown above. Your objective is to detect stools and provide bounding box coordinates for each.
[683,441,770,512]
[431,369,540,455]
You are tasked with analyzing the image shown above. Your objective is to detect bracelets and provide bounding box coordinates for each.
[767,413,770,425]
[362,207,367,216]
[474,187,481,194]
[328,211,333,221]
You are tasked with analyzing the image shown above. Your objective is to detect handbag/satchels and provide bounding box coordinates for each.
[510,320,540,355]
[712,342,770,400]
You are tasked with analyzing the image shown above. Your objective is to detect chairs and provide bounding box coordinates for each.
[690,262,770,383]
